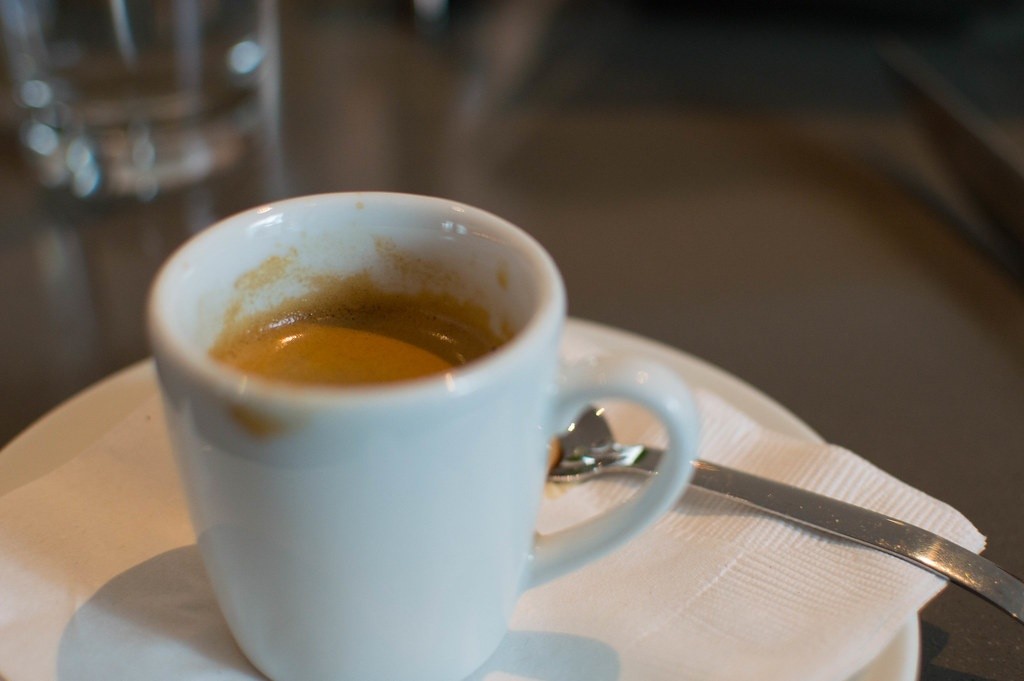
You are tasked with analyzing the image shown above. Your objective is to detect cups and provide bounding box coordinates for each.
[147,191,699,681]
[6,1,276,197]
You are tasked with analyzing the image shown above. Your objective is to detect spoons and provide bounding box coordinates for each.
[548,406,1024,621]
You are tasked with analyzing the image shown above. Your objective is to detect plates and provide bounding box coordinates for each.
[1,319,920,681]
